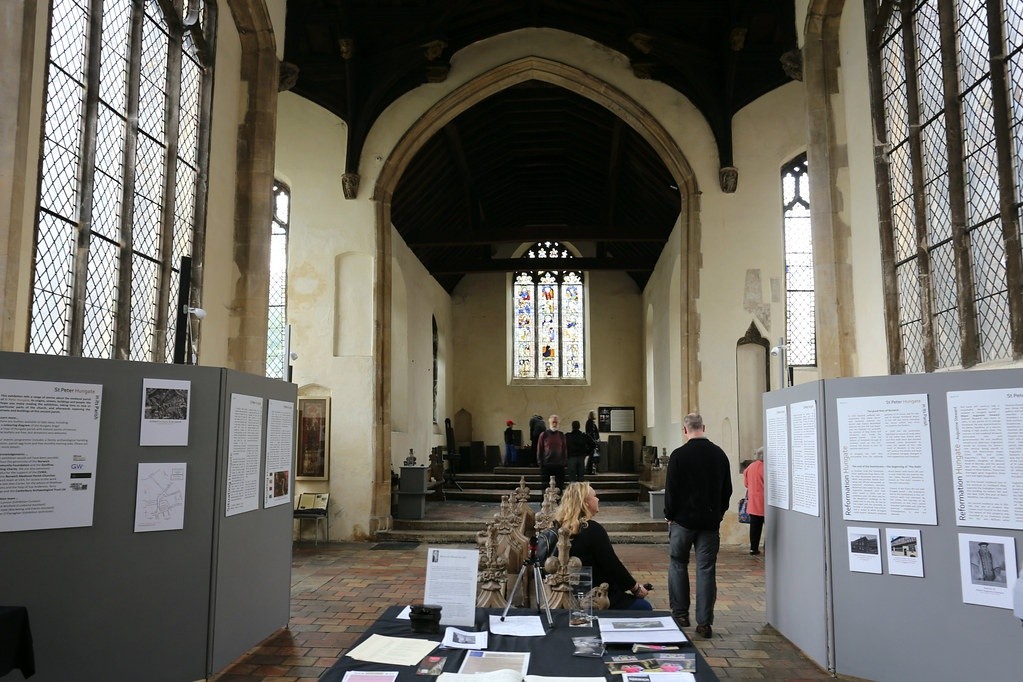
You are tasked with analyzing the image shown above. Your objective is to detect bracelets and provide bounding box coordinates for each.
[632,584,641,594]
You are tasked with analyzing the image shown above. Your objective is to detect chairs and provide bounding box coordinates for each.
[294,492,330,551]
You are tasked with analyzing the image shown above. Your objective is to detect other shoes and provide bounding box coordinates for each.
[674,617,690,627]
[750,550,761,554]
[695,625,712,639]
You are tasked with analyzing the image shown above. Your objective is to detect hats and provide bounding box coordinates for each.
[506,420,515,425]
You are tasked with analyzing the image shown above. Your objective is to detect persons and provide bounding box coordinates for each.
[529,410,600,481]
[664,413,733,639]
[971,542,1000,581]
[537,415,567,507]
[550,481,654,610]
[744,447,764,555]
[504,420,516,466]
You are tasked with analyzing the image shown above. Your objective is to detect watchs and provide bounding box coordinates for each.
[665,518,668,522]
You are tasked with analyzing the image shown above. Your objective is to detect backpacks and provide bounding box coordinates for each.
[537,520,560,580]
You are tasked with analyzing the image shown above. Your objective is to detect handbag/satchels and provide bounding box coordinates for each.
[737,490,751,522]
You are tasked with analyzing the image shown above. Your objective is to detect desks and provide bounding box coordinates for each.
[314,604,721,682]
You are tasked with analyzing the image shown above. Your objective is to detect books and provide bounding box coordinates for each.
[571,635,606,658]
[296,493,330,510]
